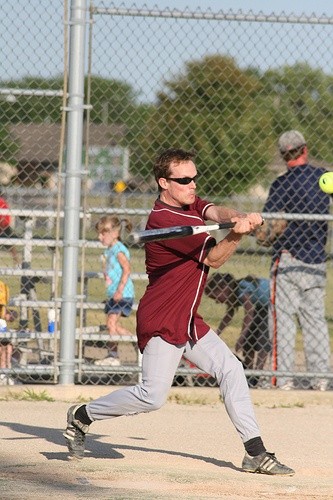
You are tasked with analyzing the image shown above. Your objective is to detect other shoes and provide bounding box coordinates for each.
[263,379,295,391]
[95,356,121,366]
[311,378,328,391]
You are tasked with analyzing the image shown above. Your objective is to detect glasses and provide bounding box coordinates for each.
[164,174,200,185]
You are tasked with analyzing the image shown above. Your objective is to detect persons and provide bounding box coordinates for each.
[0,161,23,271]
[61,149,297,476]
[250,128,333,394]
[91,215,136,369]
[0,280,18,386]
[202,269,272,388]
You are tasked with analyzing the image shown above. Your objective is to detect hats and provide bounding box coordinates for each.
[279,130,306,152]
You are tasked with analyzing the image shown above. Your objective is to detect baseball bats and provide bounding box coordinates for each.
[126,218,265,247]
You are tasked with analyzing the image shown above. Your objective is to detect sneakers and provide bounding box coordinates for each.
[63,404,92,459]
[242,451,296,477]
